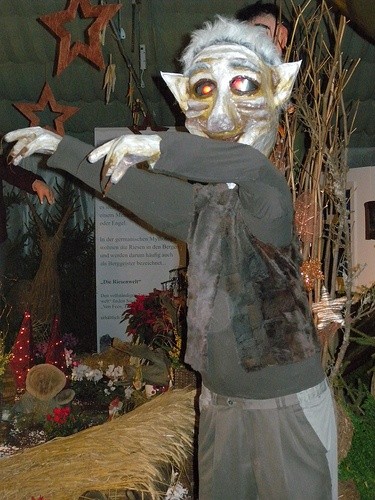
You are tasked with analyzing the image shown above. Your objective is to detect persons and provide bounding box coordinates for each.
[0,156,55,205]
[235,4,289,55]
[5,17,341,499]
